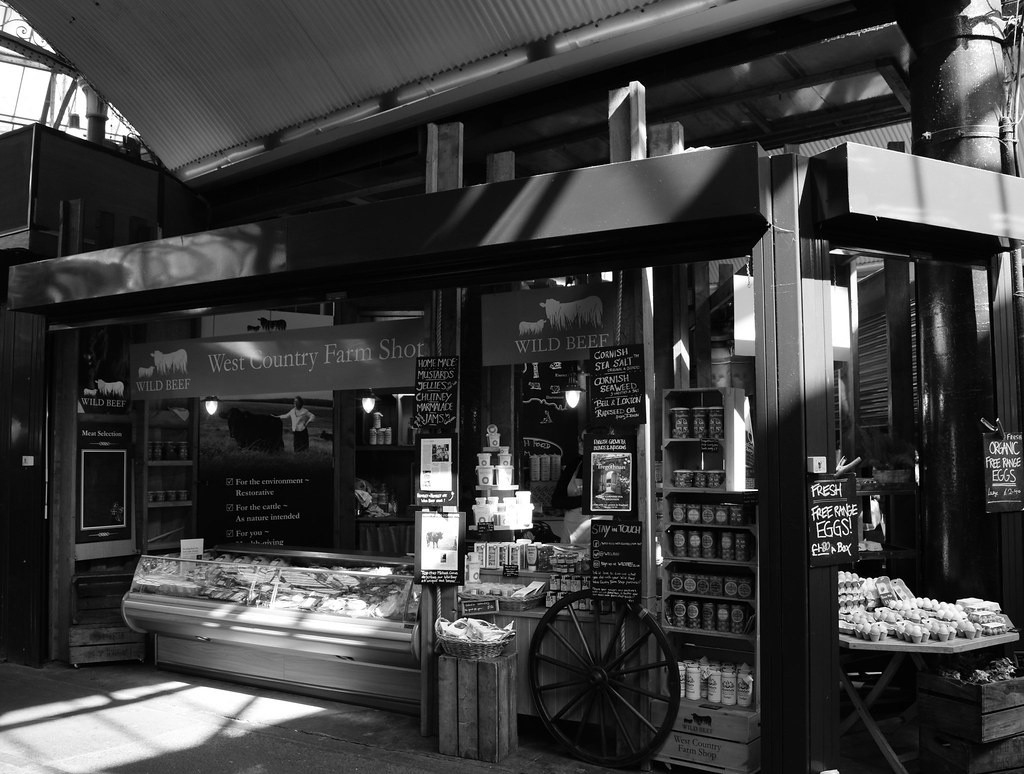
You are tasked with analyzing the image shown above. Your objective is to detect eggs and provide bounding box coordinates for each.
[838,571,1007,637]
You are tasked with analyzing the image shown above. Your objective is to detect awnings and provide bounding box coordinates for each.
[6,0,666,176]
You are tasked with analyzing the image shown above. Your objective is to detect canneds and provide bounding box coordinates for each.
[147,490,188,502]
[369,411,391,445]
[677,660,753,706]
[545,555,618,612]
[148,441,187,460]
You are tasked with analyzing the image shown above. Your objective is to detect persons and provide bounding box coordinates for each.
[269,396,316,452]
[550,426,591,547]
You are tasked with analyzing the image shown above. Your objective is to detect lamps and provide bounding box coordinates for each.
[354,390,380,413]
[201,396,223,416]
[559,367,587,408]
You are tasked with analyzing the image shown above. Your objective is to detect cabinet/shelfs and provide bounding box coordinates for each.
[640,387,761,774]
[857,490,921,599]
[332,389,415,553]
[133,397,199,555]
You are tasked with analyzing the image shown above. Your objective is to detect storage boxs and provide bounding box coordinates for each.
[912,671,1024,744]
[918,726,1024,774]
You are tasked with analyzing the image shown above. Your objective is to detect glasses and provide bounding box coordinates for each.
[578,436,583,443]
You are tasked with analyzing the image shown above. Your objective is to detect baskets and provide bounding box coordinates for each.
[434,617,517,660]
[458,592,546,612]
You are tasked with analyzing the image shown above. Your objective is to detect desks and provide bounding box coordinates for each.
[839,632,1019,774]
